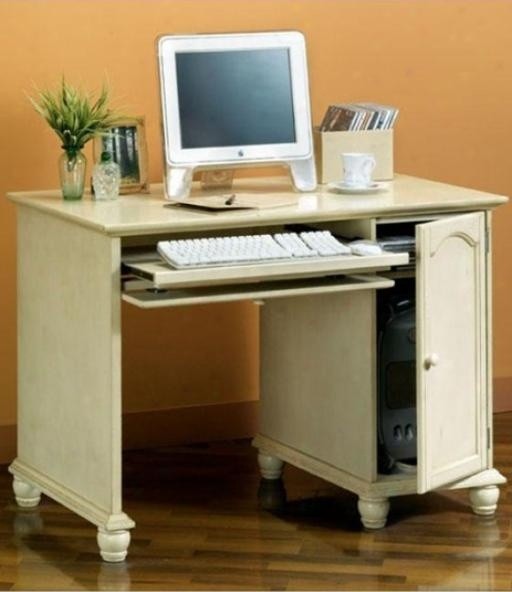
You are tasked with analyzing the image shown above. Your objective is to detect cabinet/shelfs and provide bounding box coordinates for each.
[253,207,508,530]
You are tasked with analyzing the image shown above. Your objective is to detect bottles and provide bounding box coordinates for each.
[91,153,122,201]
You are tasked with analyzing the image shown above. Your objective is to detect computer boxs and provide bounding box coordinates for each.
[376,277,417,475]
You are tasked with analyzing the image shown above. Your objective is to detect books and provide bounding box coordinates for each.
[379,235,417,269]
[320,103,400,130]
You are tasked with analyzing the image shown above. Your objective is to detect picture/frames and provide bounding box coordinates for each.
[92,116,148,193]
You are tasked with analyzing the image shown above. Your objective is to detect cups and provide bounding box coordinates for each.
[340,152,375,187]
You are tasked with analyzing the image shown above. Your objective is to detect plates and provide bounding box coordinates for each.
[327,181,392,192]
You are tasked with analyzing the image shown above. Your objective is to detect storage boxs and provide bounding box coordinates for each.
[315,127,396,184]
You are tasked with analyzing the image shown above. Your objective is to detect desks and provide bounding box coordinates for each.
[5,173,510,564]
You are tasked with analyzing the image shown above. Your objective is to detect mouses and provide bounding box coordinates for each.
[350,240,382,257]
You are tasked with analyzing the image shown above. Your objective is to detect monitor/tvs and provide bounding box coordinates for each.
[154,29,318,200]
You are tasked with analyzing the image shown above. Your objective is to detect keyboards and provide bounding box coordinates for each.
[156,230,351,270]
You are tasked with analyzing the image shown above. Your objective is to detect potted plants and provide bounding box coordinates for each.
[23,74,144,201]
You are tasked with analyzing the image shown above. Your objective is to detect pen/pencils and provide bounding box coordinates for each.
[225,193,235,206]
